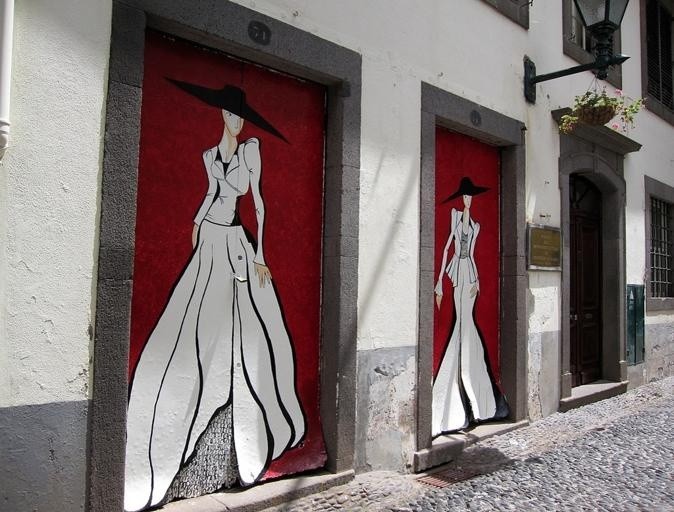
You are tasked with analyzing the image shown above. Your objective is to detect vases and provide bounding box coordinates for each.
[567,106,617,126]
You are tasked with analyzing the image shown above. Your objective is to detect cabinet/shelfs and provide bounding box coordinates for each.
[627,284,645,366]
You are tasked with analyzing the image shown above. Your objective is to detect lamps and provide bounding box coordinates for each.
[524,1,632,103]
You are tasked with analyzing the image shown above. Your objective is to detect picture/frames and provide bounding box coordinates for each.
[527,223,563,272]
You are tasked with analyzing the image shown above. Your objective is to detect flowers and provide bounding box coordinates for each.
[558,86,647,134]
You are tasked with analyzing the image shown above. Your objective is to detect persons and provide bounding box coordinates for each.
[123,76,307,512]
[431,177,510,439]
